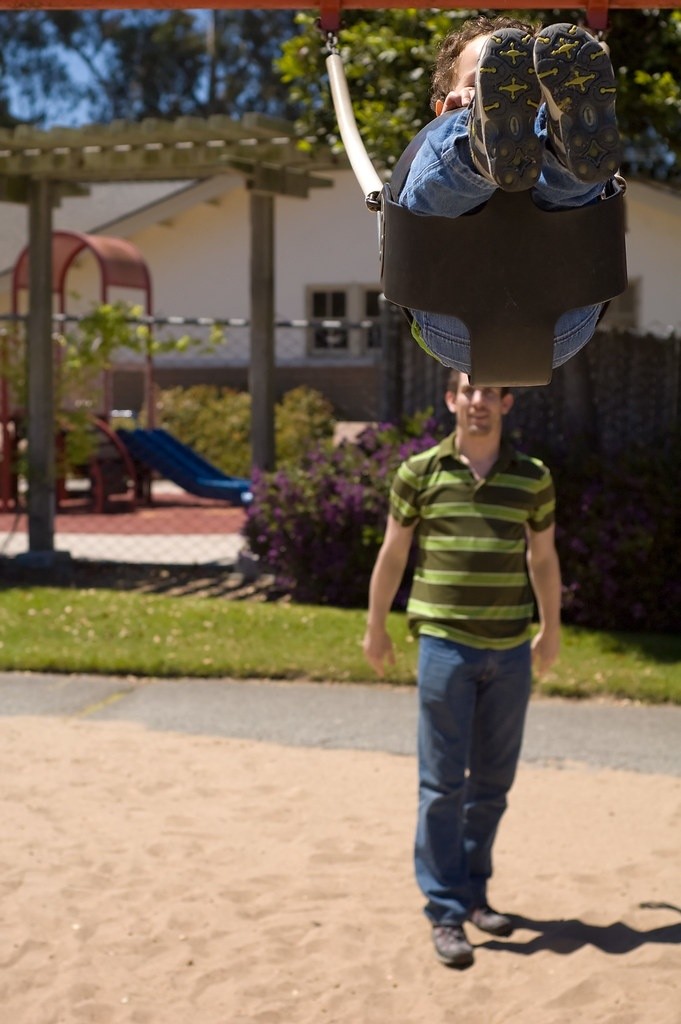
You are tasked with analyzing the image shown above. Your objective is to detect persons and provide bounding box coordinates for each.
[377,16,626,371]
[360,368,560,970]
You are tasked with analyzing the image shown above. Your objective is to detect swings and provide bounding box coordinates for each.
[311,17,632,390]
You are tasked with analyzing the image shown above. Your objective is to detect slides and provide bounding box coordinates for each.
[117,427,252,503]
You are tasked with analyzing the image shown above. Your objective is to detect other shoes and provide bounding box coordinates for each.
[431,924,475,964]
[468,901,512,935]
[533,22,622,184]
[465,28,544,192]
